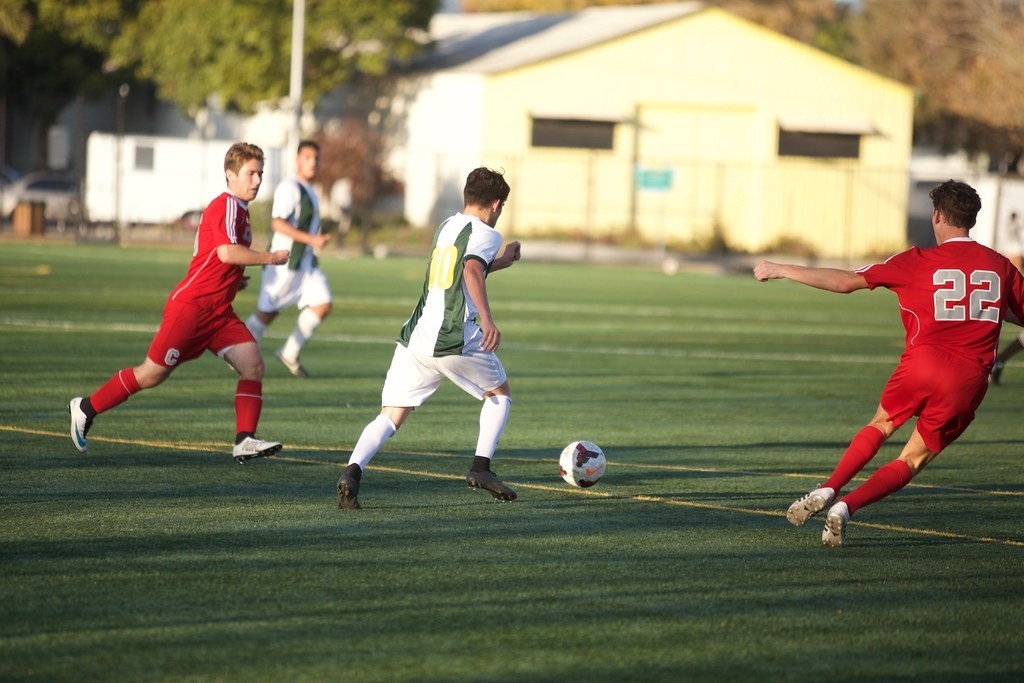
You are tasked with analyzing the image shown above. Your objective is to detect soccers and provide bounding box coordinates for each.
[558,438,608,489]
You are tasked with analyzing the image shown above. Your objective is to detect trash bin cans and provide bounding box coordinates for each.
[13,200,46,238]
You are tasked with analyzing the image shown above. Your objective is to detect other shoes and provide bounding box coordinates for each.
[991,358,1003,386]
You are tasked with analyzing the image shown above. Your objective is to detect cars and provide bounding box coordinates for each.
[0,173,85,235]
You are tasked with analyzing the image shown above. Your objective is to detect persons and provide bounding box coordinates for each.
[226,138,336,378]
[754,178,1024,550]
[987,212,1024,384]
[334,164,522,511]
[65,142,285,463]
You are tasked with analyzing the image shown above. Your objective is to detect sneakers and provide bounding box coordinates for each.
[822,502,851,548]
[466,456,518,503]
[786,487,836,527]
[232,436,283,464]
[68,397,93,454]
[275,351,309,377]
[336,463,364,508]
